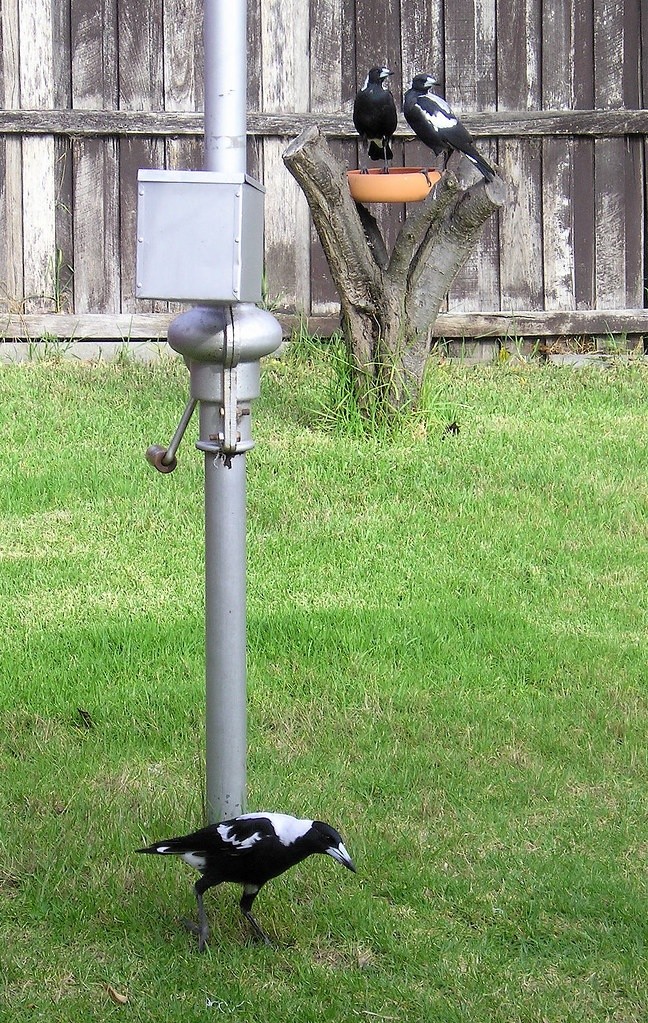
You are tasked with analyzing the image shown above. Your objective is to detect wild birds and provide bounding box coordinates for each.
[404,73,498,186]
[353,67,398,175]
[135,811,357,949]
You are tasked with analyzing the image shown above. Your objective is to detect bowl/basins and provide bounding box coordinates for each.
[345,167,442,204]
